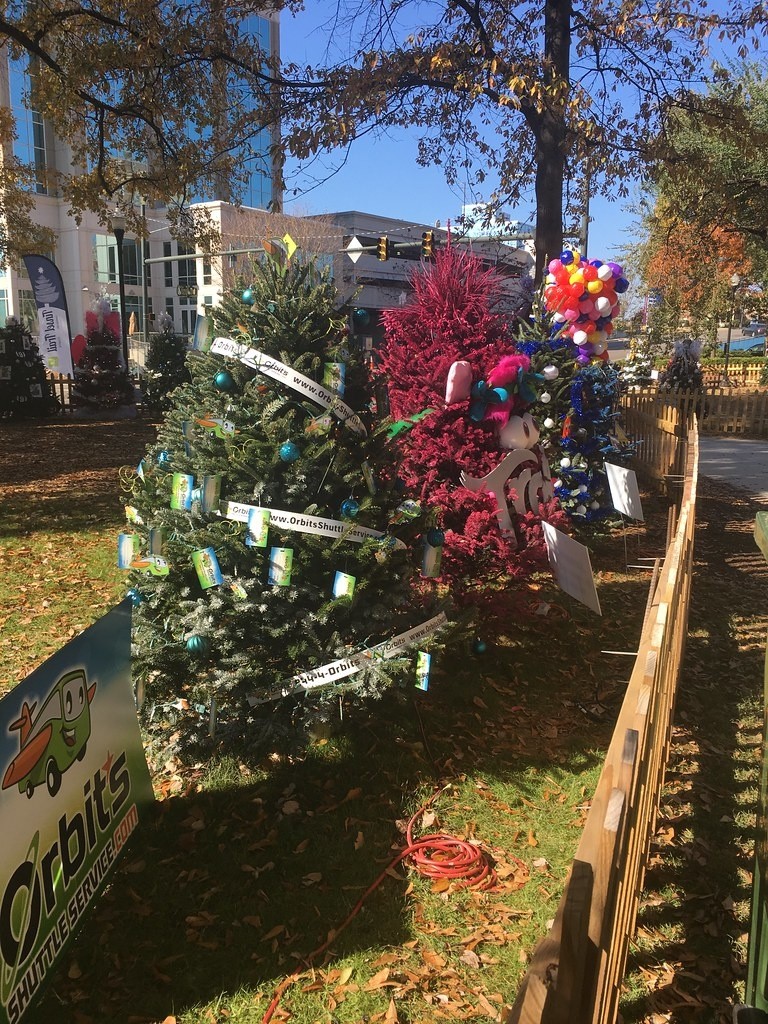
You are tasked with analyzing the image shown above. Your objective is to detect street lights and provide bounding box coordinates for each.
[720,272,742,388]
[110,202,140,419]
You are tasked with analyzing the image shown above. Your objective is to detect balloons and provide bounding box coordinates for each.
[544,251,629,366]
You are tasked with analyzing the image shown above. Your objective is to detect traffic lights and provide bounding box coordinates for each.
[423,231,435,257]
[376,237,389,263]
